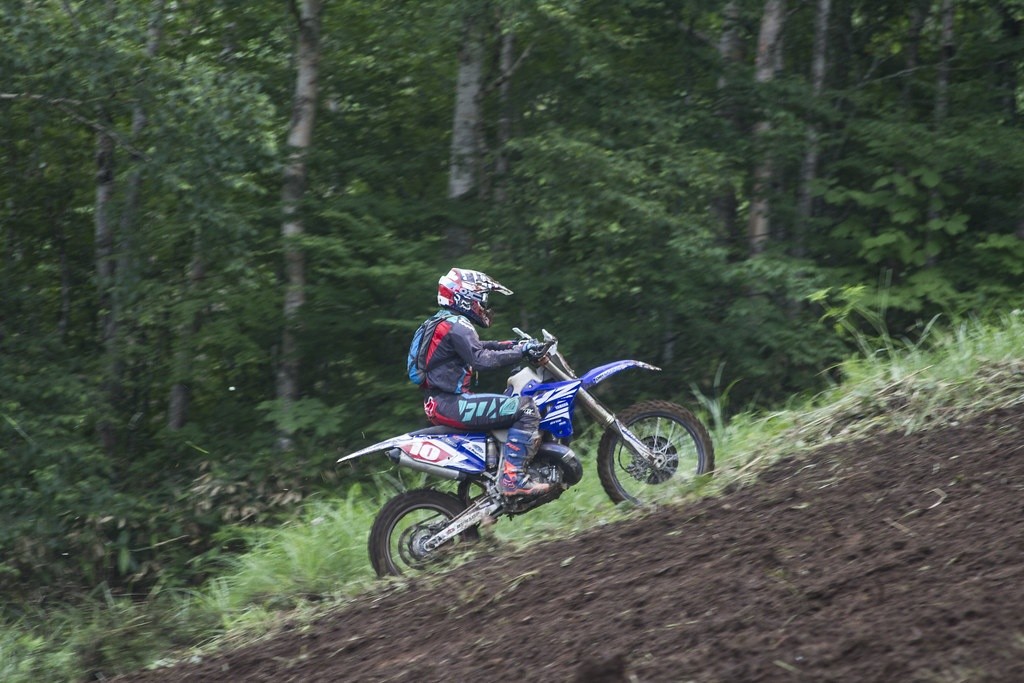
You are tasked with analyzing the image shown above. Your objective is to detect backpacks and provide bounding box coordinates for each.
[407,315,460,386]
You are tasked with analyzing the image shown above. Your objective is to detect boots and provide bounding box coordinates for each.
[497,428,549,495]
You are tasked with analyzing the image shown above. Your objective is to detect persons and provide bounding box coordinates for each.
[423,267,557,497]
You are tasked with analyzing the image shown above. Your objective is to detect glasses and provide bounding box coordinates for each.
[478,293,488,308]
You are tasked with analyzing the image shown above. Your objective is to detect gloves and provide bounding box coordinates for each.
[522,340,551,367]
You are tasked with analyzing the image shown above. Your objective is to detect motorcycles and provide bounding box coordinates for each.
[335,327,714,589]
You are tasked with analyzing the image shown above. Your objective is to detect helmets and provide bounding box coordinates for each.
[437,267,514,329]
